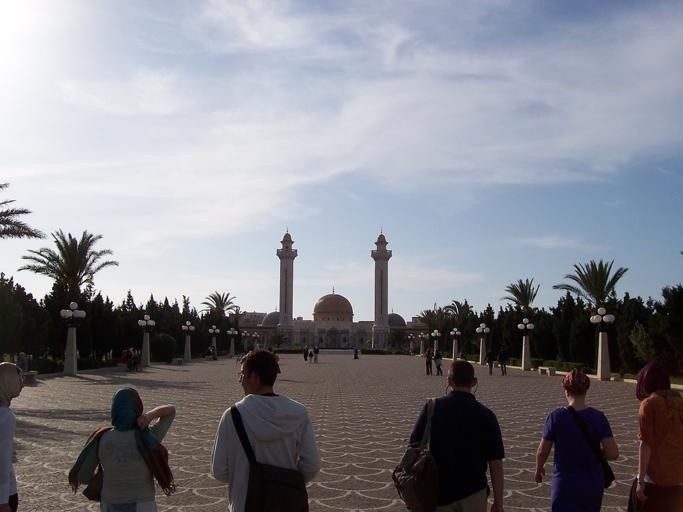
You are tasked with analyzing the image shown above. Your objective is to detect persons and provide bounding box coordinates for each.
[303,344,320,368]
[626,362,683,512]
[433,348,443,376]
[402,360,505,512]
[210,349,321,512]
[484,347,495,376]
[534,369,619,512]
[353,346,360,360]
[16,352,28,374]
[0,361,24,512]
[68,387,176,512]
[499,348,508,376]
[125,347,142,373]
[423,348,432,375]
[267,347,280,364]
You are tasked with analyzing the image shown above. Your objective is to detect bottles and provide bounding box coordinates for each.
[560,368,589,392]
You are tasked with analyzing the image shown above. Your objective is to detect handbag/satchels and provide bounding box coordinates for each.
[636,479,647,486]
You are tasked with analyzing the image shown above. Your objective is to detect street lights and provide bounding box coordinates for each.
[601,456,614,488]
[82,464,103,501]
[244,462,308,512]
[391,445,441,512]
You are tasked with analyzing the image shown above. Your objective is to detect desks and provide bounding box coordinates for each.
[518,317,534,371]
[61,302,86,376]
[450,328,461,361]
[182,321,195,362]
[476,323,490,366]
[227,328,238,355]
[431,329,441,356]
[209,325,220,349]
[408,333,415,352]
[419,333,425,354]
[252,332,259,350]
[242,331,250,353]
[138,315,155,367]
[589,307,615,381]
